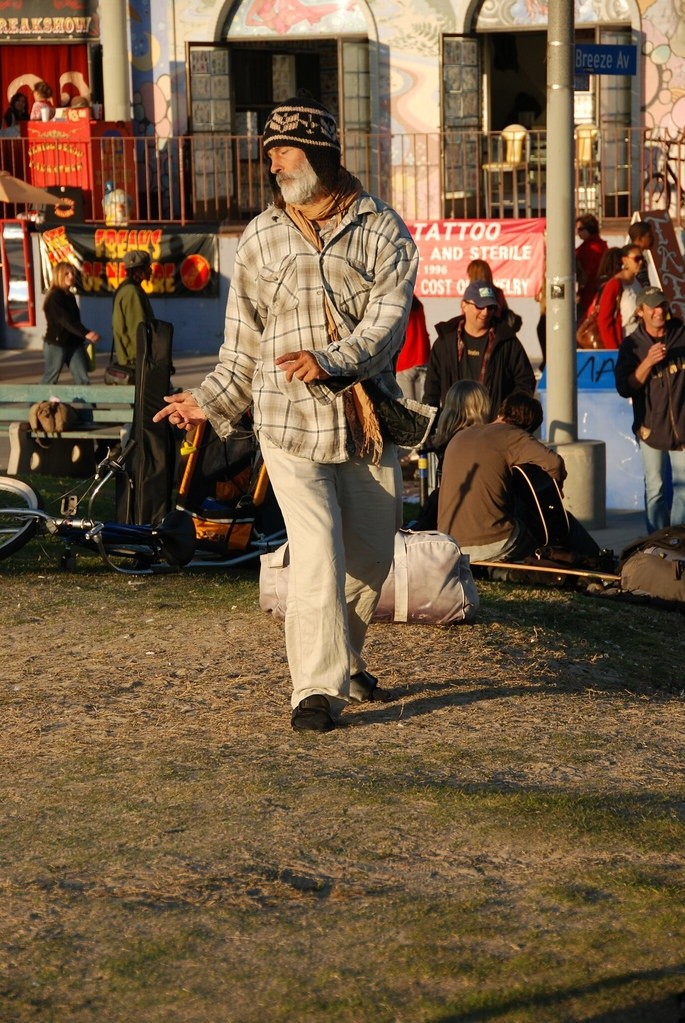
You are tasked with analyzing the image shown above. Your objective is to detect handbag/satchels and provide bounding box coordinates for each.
[619,523,685,603]
[575,279,623,349]
[28,400,76,449]
[258,525,479,625]
[81,342,94,371]
[103,364,136,386]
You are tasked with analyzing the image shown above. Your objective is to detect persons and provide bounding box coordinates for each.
[39,263,100,385]
[395,293,431,404]
[537,214,654,373]
[152,98,419,731]
[614,286,685,534]
[411,381,601,563]
[467,260,509,318]
[421,280,537,421]
[1,81,55,127]
[112,250,176,383]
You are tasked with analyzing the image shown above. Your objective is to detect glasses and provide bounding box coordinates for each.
[627,254,644,263]
[465,301,494,311]
[577,226,587,232]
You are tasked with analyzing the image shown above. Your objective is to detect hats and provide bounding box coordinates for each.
[463,279,497,306]
[635,285,668,307]
[263,88,340,188]
[124,249,150,269]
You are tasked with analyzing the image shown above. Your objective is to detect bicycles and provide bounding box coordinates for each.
[0,437,198,578]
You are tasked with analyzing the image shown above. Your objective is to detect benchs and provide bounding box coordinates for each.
[0,381,182,480]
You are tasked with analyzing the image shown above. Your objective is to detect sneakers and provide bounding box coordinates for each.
[347,671,378,702]
[291,693,334,731]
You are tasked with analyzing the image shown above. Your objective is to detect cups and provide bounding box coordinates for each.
[93,104,102,119]
[41,108,50,122]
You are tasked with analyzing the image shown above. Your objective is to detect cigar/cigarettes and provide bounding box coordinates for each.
[662,347,666,350]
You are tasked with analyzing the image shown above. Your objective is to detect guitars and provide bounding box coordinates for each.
[512,463,570,547]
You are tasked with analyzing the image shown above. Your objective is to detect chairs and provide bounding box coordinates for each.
[573,124,604,218]
[482,125,531,219]
